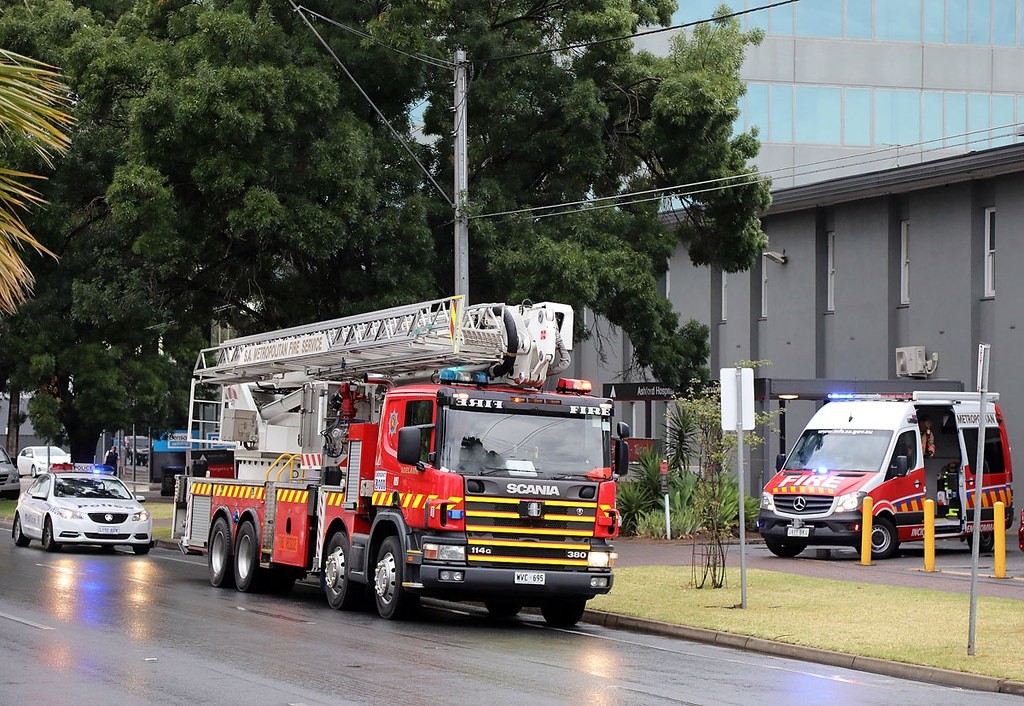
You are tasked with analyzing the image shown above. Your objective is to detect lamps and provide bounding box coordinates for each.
[763,251,786,263]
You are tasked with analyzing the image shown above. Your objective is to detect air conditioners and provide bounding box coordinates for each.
[896,346,926,374]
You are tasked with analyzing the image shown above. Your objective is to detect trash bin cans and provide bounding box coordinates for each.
[161,466,183,496]
[136,453,147,466]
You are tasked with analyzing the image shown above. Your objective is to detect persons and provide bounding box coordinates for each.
[906,415,935,458]
[104,446,120,477]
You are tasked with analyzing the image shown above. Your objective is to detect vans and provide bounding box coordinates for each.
[756,390,1016,564]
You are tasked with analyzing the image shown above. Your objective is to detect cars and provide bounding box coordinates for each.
[0,443,21,499]
[11,463,153,555]
[112,435,150,468]
[17,445,72,478]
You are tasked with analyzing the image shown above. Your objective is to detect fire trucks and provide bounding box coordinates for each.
[170,294,631,629]
